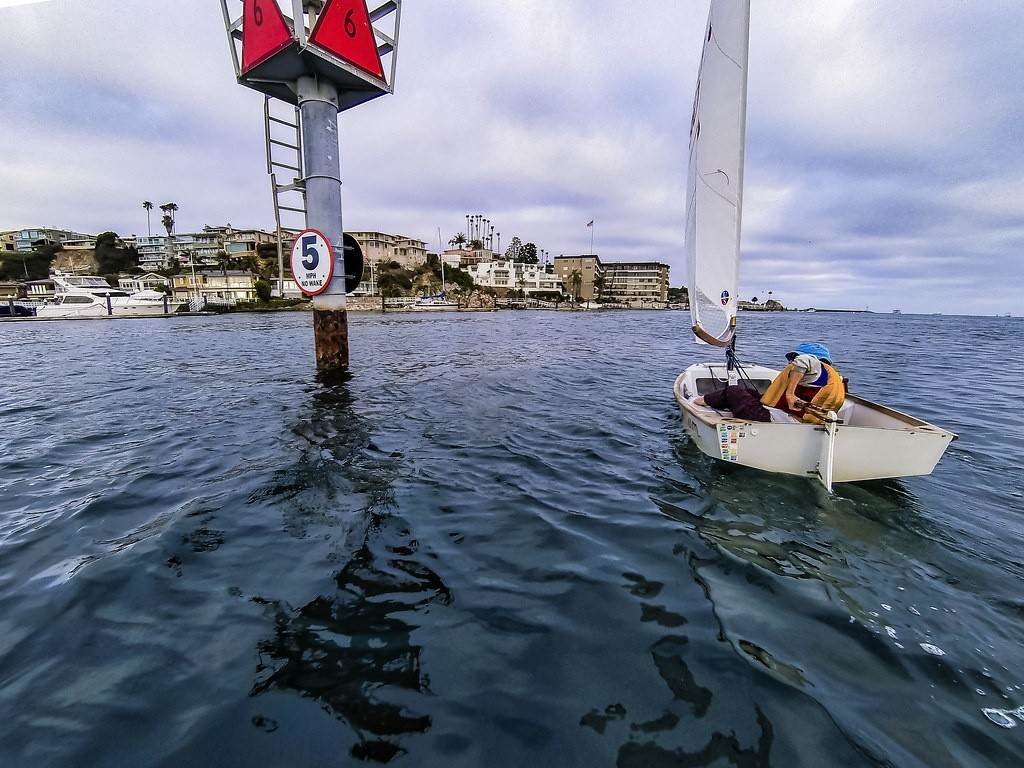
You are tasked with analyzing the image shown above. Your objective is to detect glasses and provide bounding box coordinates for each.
[787,355,795,361]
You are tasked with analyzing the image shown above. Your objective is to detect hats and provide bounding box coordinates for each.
[785,342,833,366]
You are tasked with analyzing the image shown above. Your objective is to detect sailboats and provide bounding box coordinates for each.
[412,227,466,311]
[671,0,961,496]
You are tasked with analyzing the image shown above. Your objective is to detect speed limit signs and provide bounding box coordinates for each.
[288,228,334,297]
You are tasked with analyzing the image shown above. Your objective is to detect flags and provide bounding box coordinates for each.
[587,220,593,227]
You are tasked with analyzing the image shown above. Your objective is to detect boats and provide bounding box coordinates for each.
[34,276,187,319]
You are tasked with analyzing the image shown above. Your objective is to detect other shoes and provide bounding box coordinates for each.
[684,384,692,400]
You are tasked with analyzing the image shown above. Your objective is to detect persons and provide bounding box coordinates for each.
[680,341,847,425]
